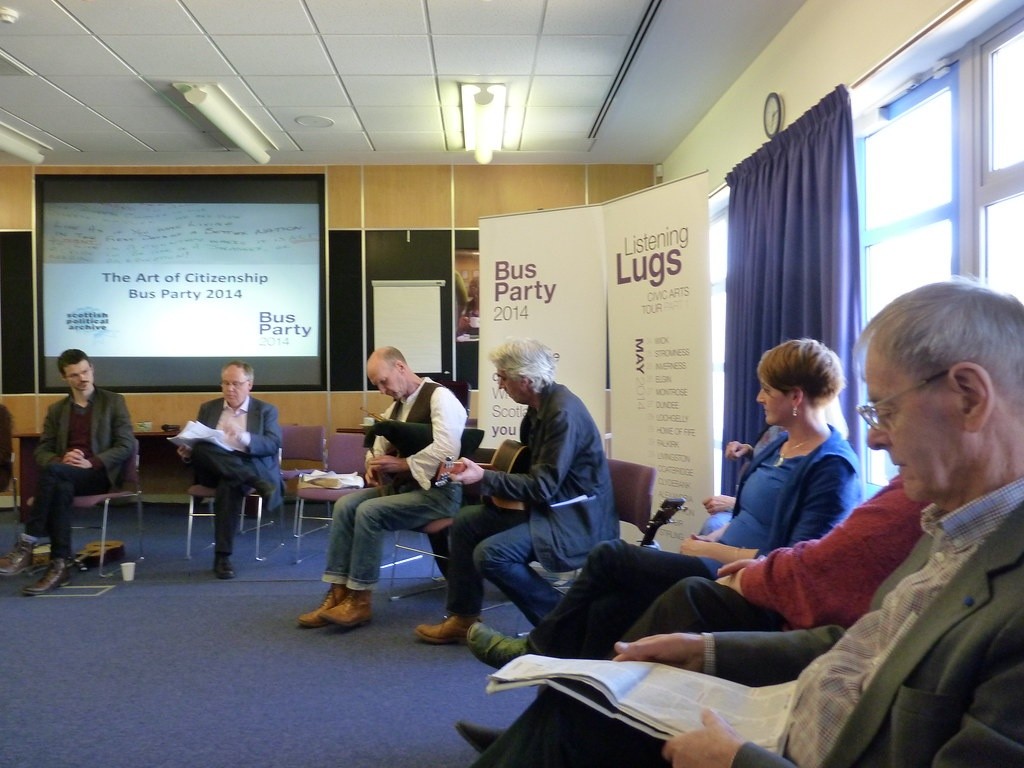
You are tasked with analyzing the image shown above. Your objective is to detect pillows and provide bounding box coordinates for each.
[364,419,486,460]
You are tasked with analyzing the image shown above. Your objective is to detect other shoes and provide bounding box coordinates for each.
[455,721,508,753]
[467,621,544,669]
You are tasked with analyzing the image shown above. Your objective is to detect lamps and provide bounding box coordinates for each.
[172,81,280,166]
[0,121,53,164]
[461,81,507,165]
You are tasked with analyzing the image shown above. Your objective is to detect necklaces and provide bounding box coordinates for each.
[773,441,806,467]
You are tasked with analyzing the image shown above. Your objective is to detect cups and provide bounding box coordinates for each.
[363,417,376,425]
[121,562,136,581]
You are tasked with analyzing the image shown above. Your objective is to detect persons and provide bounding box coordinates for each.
[413,337,618,644]
[465,280,1024,768]
[467,337,861,668]
[457,476,935,754]
[178,360,283,579]
[297,345,468,627]
[0,349,135,596]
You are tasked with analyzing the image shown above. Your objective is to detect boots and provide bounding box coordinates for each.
[319,588,372,627]
[296,582,349,627]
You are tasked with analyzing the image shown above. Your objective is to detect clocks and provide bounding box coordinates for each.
[763,92,784,140]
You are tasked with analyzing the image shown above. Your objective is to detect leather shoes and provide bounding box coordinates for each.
[255,480,277,499]
[0,537,33,576]
[23,557,73,595]
[415,613,477,644]
[214,556,235,578]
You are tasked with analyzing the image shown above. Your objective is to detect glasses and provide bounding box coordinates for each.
[857,367,942,432]
[219,380,249,389]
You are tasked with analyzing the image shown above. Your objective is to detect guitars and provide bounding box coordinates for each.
[72,539,126,570]
[432,438,527,511]
[638,496,690,547]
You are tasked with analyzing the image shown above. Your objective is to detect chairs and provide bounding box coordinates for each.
[481,457,658,639]
[185,425,286,562]
[292,432,370,566]
[25,438,146,579]
[380,516,458,602]
[240,425,331,538]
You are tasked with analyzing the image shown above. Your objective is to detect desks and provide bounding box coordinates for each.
[11,423,297,524]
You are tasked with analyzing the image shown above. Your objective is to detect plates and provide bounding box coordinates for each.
[360,423,373,428]
